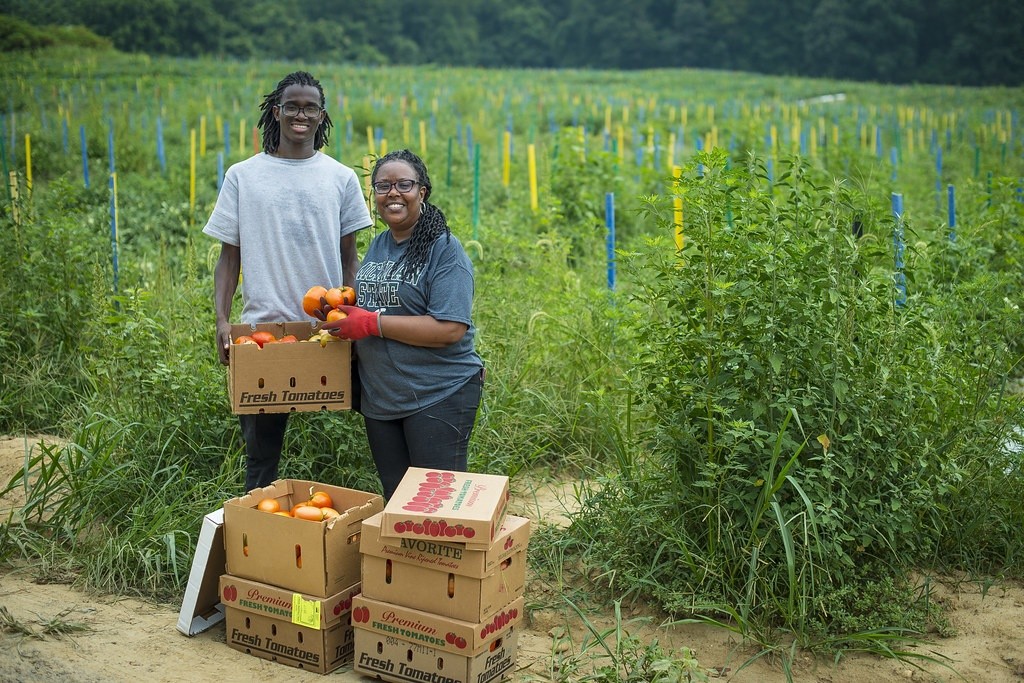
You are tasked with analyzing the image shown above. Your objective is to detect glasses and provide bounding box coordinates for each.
[277,103,324,118]
[373,180,424,194]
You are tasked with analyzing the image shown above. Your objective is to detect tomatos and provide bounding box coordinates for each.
[233,286,530,683]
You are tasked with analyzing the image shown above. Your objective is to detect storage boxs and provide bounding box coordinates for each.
[224,321,351,415]
[177,466,530,683]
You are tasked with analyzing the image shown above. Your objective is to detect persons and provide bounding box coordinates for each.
[313,151,486,502]
[201,71,374,493]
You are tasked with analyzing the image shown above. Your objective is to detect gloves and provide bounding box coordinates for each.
[322,305,384,340]
[314,296,348,321]
